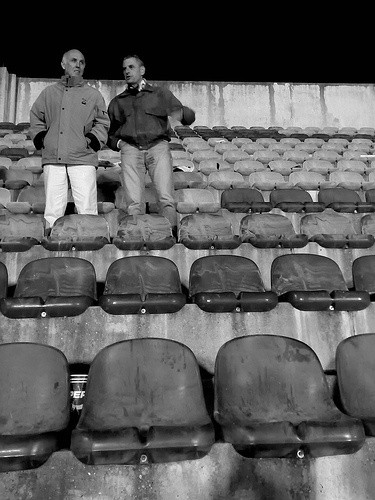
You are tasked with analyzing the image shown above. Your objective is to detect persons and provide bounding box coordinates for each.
[107,55,196,243]
[28,49,110,230]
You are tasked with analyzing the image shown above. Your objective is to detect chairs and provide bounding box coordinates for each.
[0,118,374,473]
